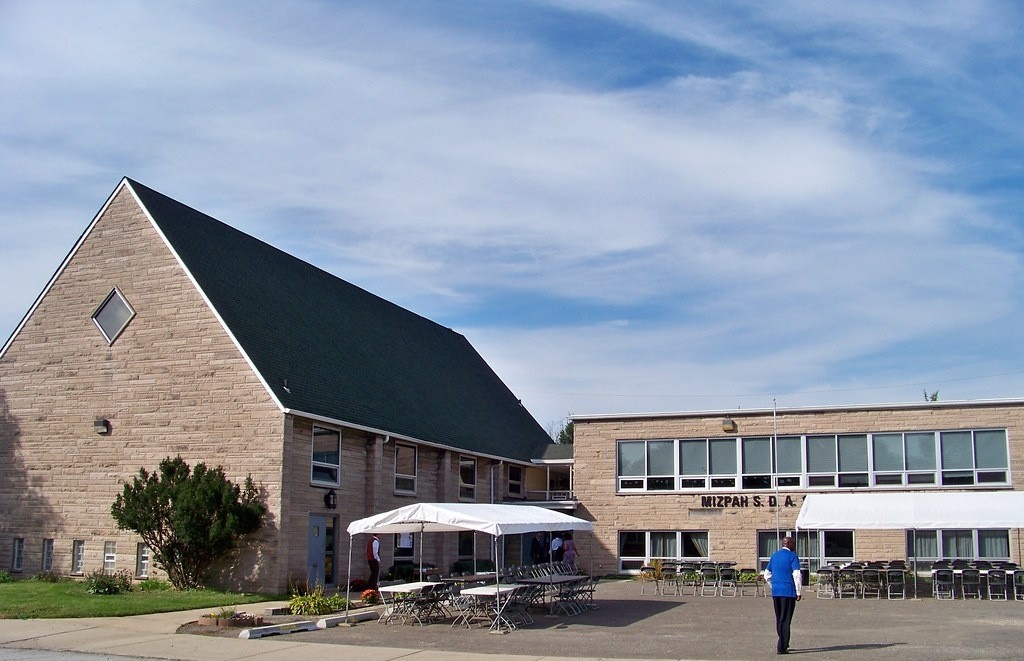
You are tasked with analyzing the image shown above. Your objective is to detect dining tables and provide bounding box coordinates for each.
[376,559,600,633]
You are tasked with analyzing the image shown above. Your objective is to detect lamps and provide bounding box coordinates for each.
[93,420,109,435]
[722,419,733,431]
[325,488,337,509]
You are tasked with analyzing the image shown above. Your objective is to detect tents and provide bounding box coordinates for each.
[339,501,595,634]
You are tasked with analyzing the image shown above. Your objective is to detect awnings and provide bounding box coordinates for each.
[796,491,1024,531]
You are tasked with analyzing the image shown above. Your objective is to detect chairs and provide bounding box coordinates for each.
[931,560,1024,601]
[641,561,769,598]
[815,559,911,600]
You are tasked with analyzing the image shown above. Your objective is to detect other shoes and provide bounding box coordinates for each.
[776,651,789,654]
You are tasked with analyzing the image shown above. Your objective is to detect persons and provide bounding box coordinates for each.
[530,531,581,571]
[365,533,383,589]
[764,536,803,655]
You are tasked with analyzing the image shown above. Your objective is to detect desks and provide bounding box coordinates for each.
[515,574,590,615]
[412,567,438,582]
[460,582,532,630]
[378,581,441,625]
[440,573,513,607]
[815,568,1015,597]
